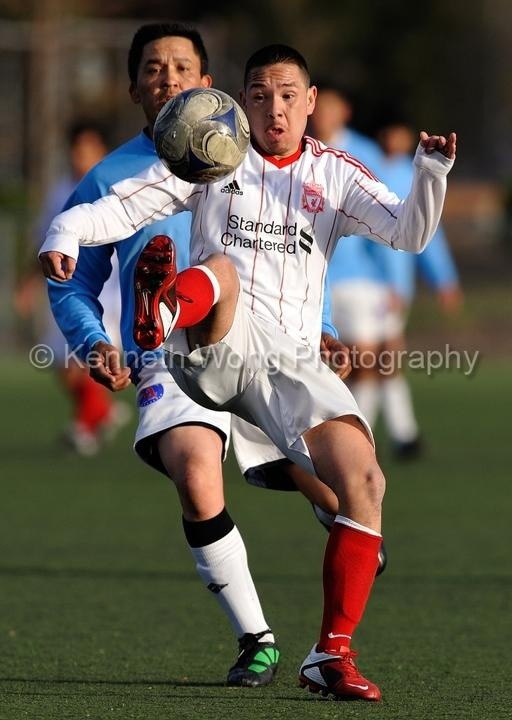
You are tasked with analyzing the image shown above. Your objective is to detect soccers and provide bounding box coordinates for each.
[154,86,250,184]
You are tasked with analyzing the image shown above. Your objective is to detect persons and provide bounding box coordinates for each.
[16,119,112,458]
[305,84,402,434]
[46,20,354,686]
[373,110,465,461]
[38,43,457,702]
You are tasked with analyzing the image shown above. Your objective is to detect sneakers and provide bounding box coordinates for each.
[133,234,181,350]
[297,644,379,699]
[311,501,387,576]
[227,633,280,685]
